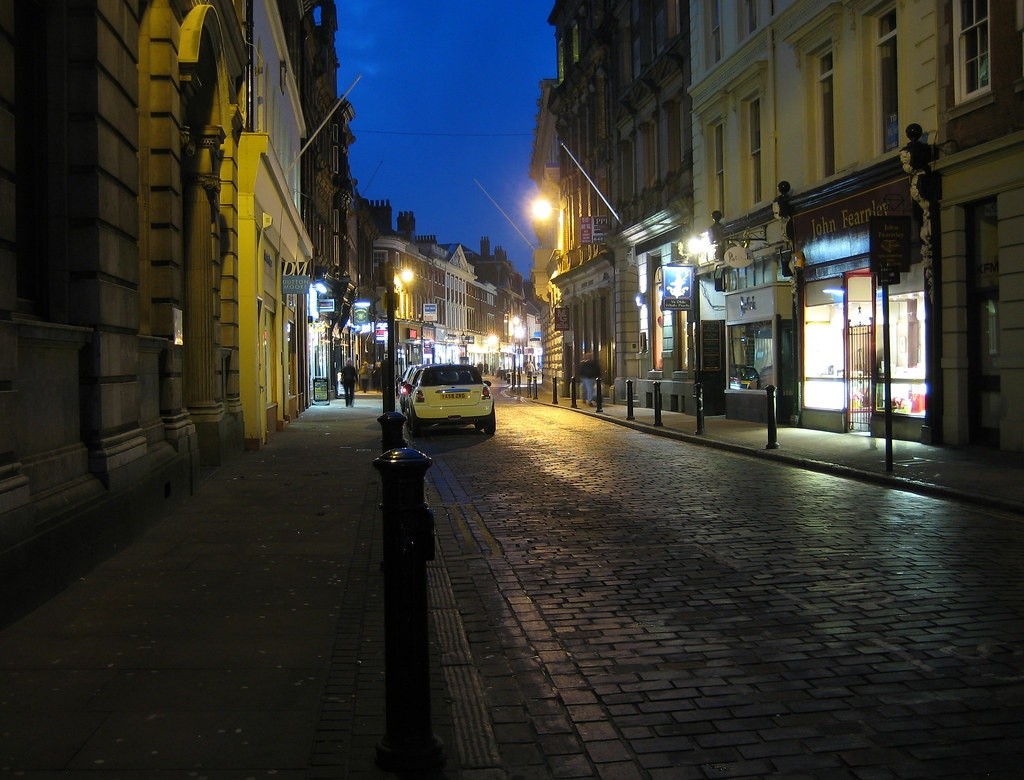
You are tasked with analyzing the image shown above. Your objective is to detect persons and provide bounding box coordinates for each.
[341,359,358,407]
[578,352,597,406]
[372,360,382,393]
[526,361,535,376]
[358,361,371,392]
[477,362,483,372]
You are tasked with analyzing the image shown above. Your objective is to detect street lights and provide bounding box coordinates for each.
[460,331,469,357]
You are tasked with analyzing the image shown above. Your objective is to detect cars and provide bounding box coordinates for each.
[395,365,424,414]
[401,363,495,435]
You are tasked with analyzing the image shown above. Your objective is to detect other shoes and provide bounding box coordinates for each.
[582,399,586,403]
[589,403,595,407]
